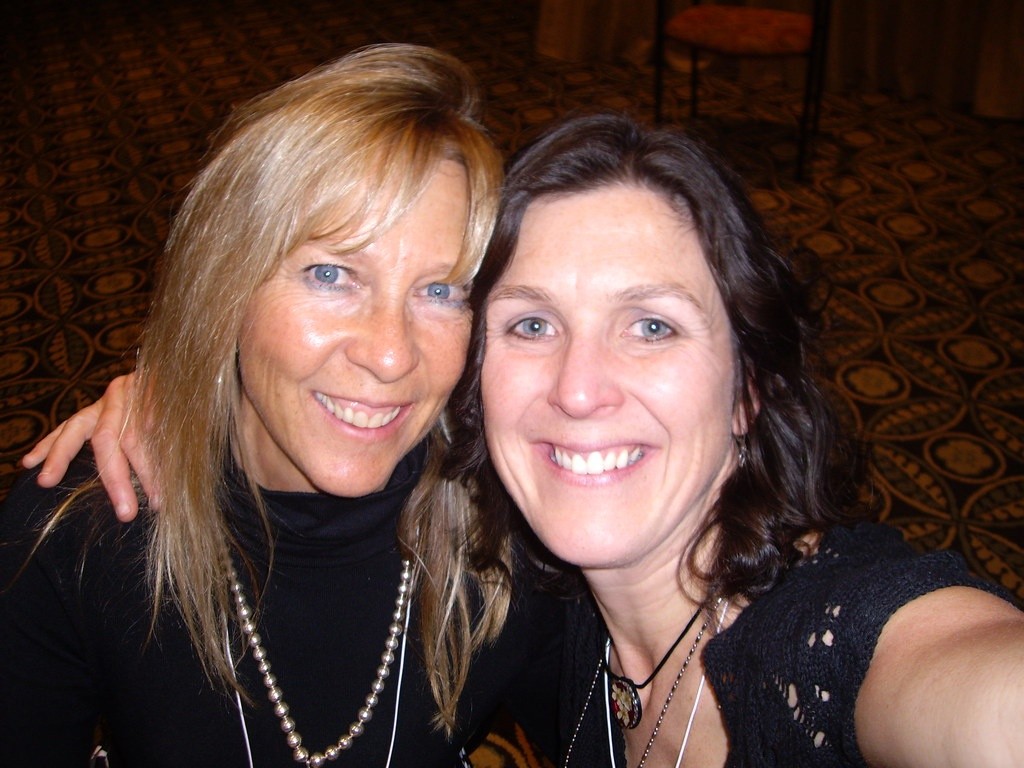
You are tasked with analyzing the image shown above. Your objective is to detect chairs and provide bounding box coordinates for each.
[653,0,832,182]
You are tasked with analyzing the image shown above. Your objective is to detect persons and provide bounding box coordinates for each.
[0,42,1024,768]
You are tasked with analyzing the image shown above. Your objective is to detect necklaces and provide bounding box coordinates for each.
[601,602,706,729]
[220,523,419,768]
[215,517,411,768]
[605,601,728,768]
[565,598,722,768]
